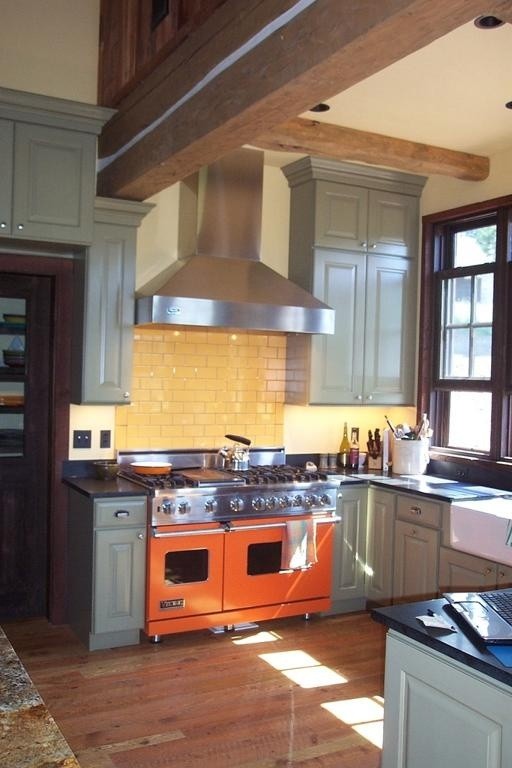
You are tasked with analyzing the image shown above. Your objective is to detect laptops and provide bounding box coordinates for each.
[442,592,512,646]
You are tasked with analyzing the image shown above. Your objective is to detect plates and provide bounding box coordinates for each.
[0,397,24,406]
[130,462,172,475]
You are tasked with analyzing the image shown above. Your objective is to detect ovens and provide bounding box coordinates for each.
[143,523,224,637]
[223,511,338,625]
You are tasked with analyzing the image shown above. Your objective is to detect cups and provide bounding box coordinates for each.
[358,453,366,470]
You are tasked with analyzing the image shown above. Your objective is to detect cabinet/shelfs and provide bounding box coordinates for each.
[366,485,396,611]
[68,487,148,651]
[280,155,429,407]
[392,490,441,610]
[381,629,512,768]
[72,195,157,405]
[311,484,369,618]
[1,85,118,252]
[438,501,512,598]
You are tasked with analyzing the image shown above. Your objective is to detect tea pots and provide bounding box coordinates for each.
[219,434,251,471]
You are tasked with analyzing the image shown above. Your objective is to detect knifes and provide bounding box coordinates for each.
[367,428,380,454]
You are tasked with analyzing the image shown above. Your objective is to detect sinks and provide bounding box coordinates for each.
[451,496,512,566]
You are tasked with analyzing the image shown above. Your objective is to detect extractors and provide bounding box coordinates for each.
[135,141,337,337]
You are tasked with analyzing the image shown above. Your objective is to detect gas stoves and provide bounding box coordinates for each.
[117,464,343,526]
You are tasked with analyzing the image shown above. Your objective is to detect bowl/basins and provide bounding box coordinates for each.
[93,461,121,481]
[2,313,26,324]
[2,349,25,367]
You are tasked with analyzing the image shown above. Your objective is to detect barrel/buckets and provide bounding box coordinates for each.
[392,437,430,474]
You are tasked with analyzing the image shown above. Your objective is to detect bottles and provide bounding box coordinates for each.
[339,422,351,473]
[348,427,360,474]
